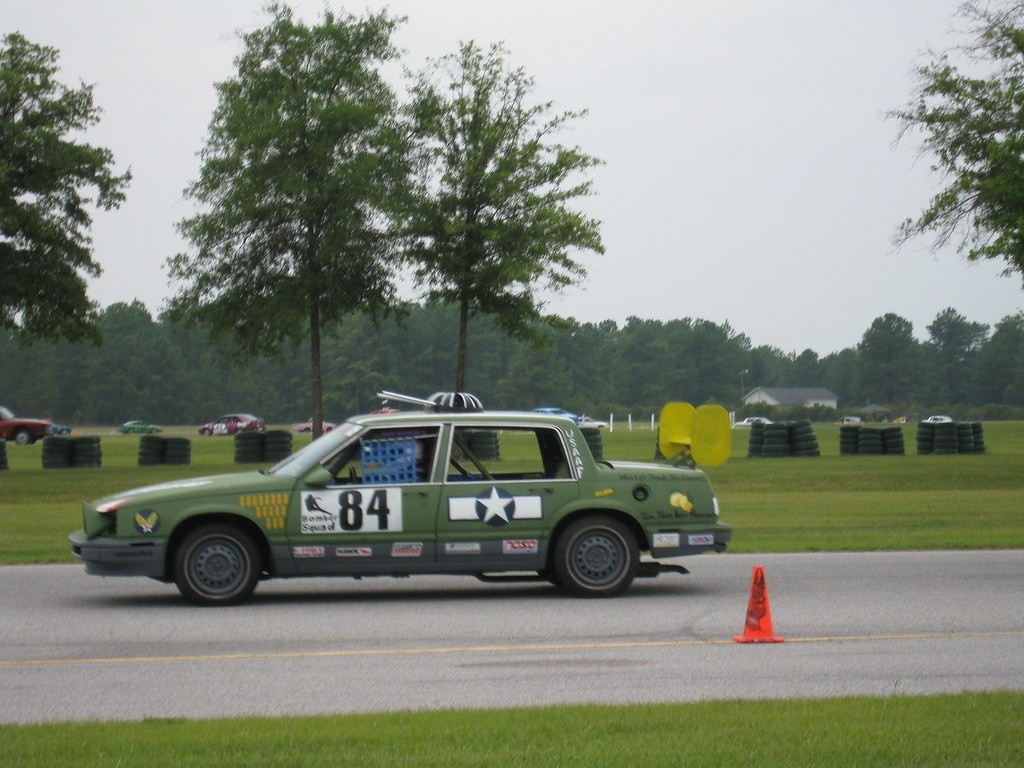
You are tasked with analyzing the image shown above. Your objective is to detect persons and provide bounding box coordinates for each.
[384,439,426,479]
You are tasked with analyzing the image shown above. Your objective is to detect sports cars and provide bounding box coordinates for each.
[198,413,266,435]
[293,417,335,434]
[116,419,164,434]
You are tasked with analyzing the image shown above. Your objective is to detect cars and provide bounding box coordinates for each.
[734,418,774,426]
[0,406,54,445]
[532,407,579,426]
[51,423,71,437]
[920,416,952,424]
[66,391,736,609]
[577,416,609,429]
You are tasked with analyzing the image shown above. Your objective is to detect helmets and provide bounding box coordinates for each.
[376,439,422,471]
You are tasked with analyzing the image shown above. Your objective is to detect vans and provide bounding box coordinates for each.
[836,417,861,424]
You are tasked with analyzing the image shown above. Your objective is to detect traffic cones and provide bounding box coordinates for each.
[734,566,786,642]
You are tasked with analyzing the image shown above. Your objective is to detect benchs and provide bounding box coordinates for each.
[546,448,570,480]
